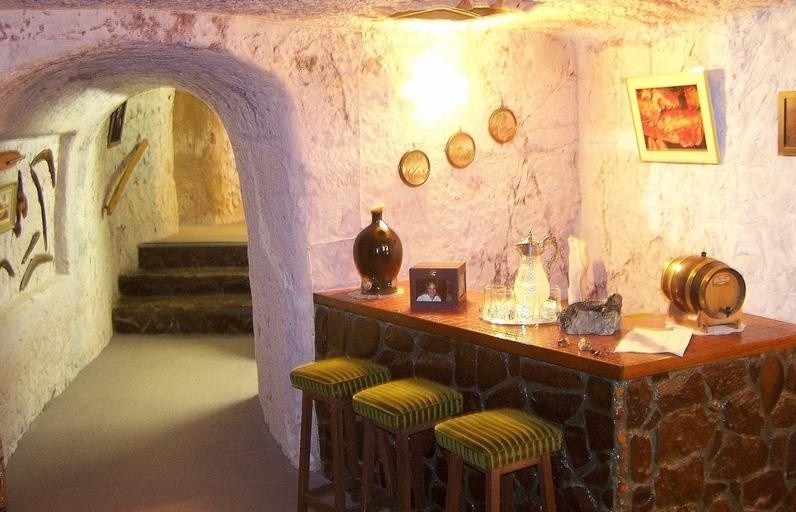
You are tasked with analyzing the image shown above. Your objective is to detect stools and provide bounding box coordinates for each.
[432,406,563,510]
[349,375,465,510]
[288,354,395,510]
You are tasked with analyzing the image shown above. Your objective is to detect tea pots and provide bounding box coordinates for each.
[512,229,561,308]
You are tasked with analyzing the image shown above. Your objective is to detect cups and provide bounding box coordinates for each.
[483,285,561,324]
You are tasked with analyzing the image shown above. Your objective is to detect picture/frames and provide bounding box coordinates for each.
[108,102,127,147]
[624,68,720,164]
[777,90,796,155]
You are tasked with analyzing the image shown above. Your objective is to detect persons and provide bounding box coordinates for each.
[417,280,441,301]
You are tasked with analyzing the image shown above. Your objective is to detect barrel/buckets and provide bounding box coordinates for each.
[660,252,746,320]
